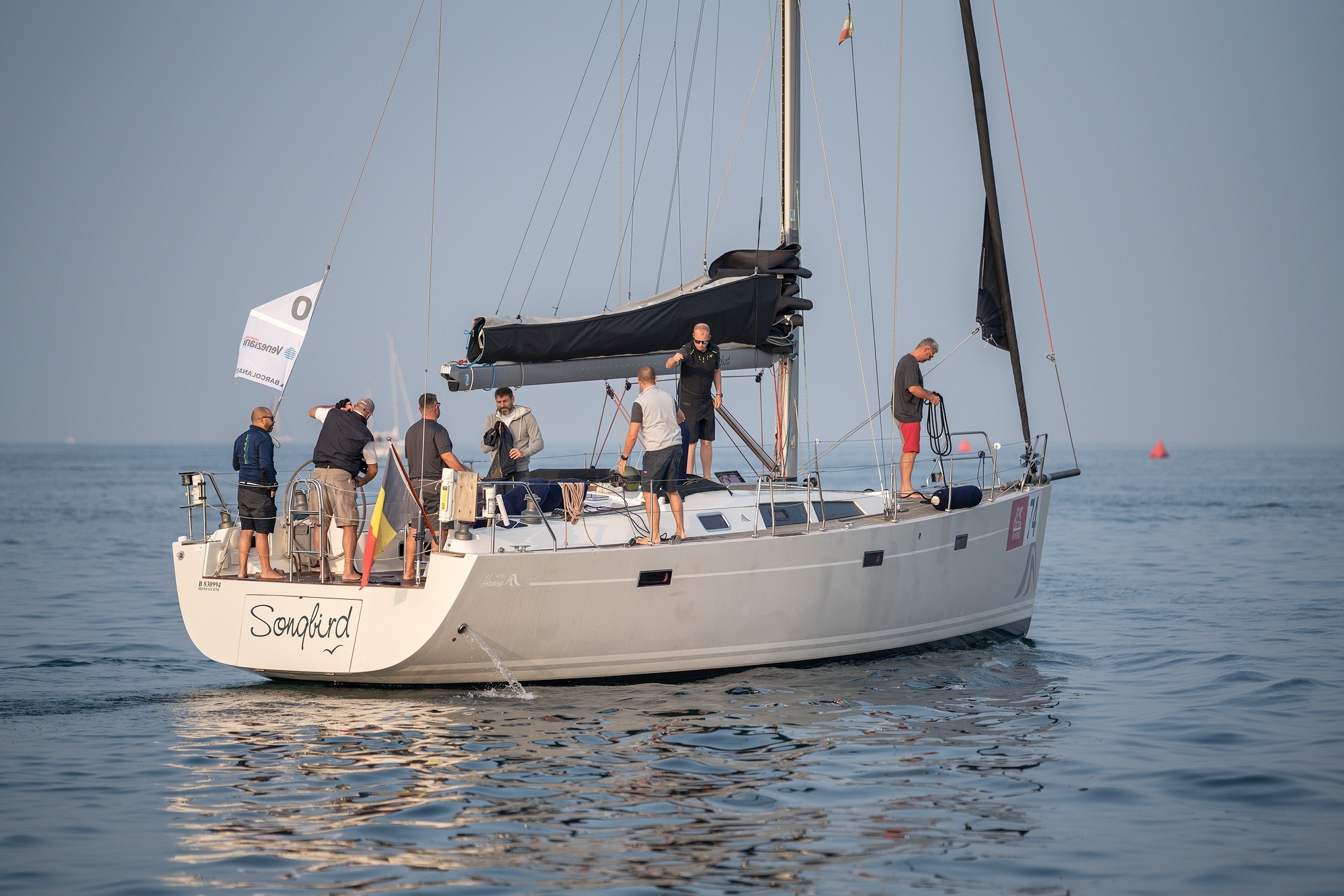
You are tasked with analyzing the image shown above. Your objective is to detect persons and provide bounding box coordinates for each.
[666,323,723,481]
[481,388,544,498]
[233,407,288,578]
[619,366,686,546]
[891,338,940,499]
[403,393,467,582]
[309,397,378,583]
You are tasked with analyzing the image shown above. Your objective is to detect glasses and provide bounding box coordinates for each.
[258,415,274,422]
[693,338,710,344]
[924,346,934,358]
[435,402,441,407]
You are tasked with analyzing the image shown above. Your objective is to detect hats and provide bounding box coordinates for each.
[356,397,375,416]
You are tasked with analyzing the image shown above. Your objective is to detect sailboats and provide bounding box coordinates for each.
[171,0,1084,690]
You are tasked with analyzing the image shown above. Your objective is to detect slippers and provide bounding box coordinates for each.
[319,575,335,581]
[343,575,363,582]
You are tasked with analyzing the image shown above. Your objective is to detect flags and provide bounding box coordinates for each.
[838,8,853,45]
[358,443,421,590]
[234,278,324,392]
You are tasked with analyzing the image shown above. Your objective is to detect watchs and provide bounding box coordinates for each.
[621,454,628,461]
[716,393,723,398]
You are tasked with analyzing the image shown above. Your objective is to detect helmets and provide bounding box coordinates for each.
[614,466,641,492]
